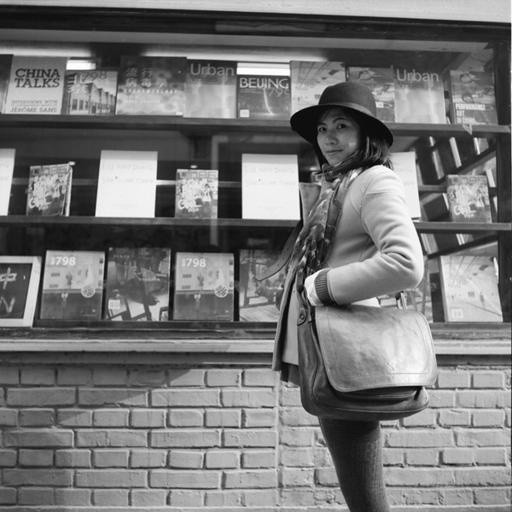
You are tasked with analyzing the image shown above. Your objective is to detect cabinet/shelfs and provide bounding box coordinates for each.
[0,4,512,340]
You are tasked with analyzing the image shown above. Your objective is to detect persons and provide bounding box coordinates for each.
[270,80,427,512]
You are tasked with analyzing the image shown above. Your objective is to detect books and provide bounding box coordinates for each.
[0,144,320,220]
[0,242,286,324]
[0,53,501,125]
[385,149,502,324]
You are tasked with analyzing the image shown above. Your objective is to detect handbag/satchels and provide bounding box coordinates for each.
[297,304,439,422]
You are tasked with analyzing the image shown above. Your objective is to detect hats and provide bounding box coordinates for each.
[289,82,394,150]
